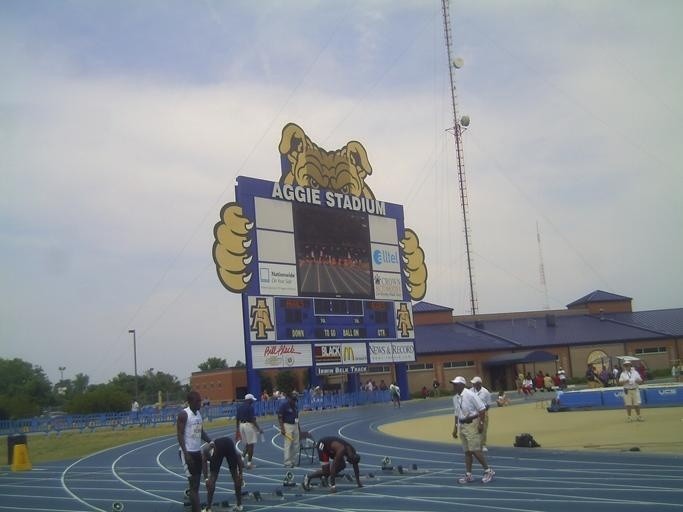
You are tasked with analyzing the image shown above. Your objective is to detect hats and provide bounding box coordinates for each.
[450,376,466,384]
[245,394,257,401]
[623,361,631,365]
[470,377,482,384]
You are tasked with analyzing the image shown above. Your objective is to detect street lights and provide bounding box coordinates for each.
[57,365,67,380]
[127,329,138,400]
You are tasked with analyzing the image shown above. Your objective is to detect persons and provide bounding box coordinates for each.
[131,399,140,412]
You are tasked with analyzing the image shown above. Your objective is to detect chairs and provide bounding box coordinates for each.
[0,388,394,434]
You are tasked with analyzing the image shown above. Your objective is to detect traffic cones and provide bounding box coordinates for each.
[8,443,32,472]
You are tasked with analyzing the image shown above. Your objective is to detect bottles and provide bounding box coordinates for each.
[259,431,266,443]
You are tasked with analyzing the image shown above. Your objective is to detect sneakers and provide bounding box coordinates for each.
[303,473,311,489]
[482,469,495,483]
[459,474,474,483]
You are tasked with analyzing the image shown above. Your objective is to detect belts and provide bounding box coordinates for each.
[459,415,478,423]
